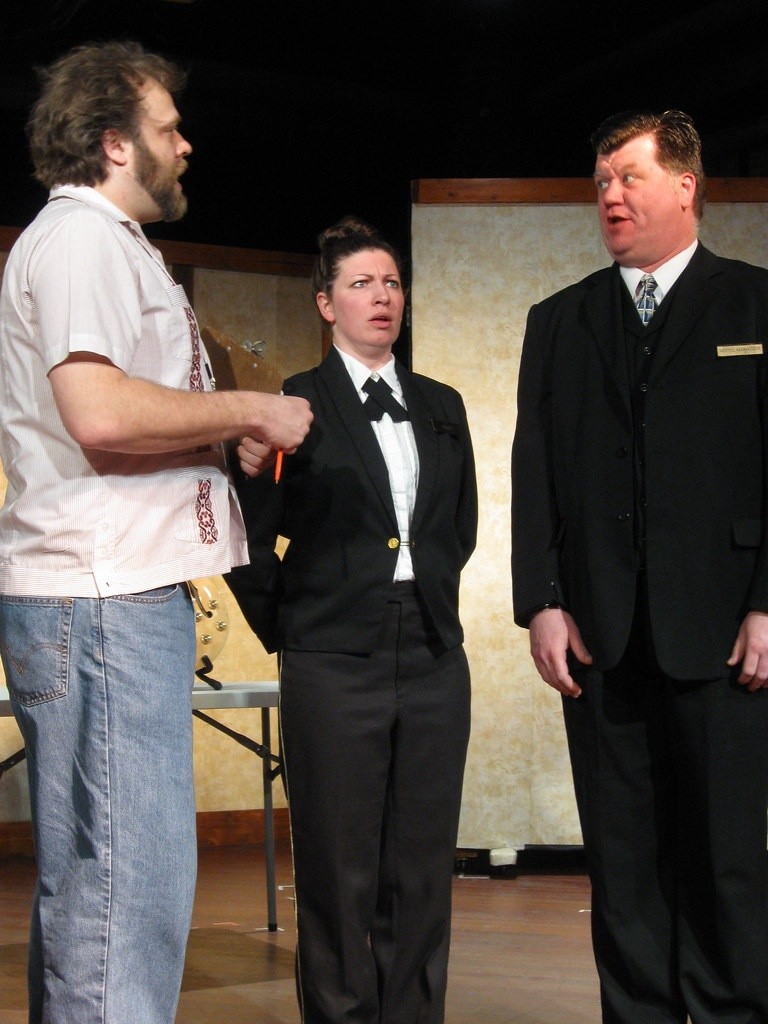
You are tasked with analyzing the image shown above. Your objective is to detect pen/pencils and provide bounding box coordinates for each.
[274,449,283,484]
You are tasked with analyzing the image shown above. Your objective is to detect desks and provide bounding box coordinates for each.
[0,680,279,932]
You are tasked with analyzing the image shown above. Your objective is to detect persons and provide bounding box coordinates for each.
[511,105,767,1024]
[0,42,314,1024]
[221,222,479,1024]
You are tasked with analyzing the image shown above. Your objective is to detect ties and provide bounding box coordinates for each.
[635,273,658,327]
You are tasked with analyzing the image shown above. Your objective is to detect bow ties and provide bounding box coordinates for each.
[360,377,411,423]
